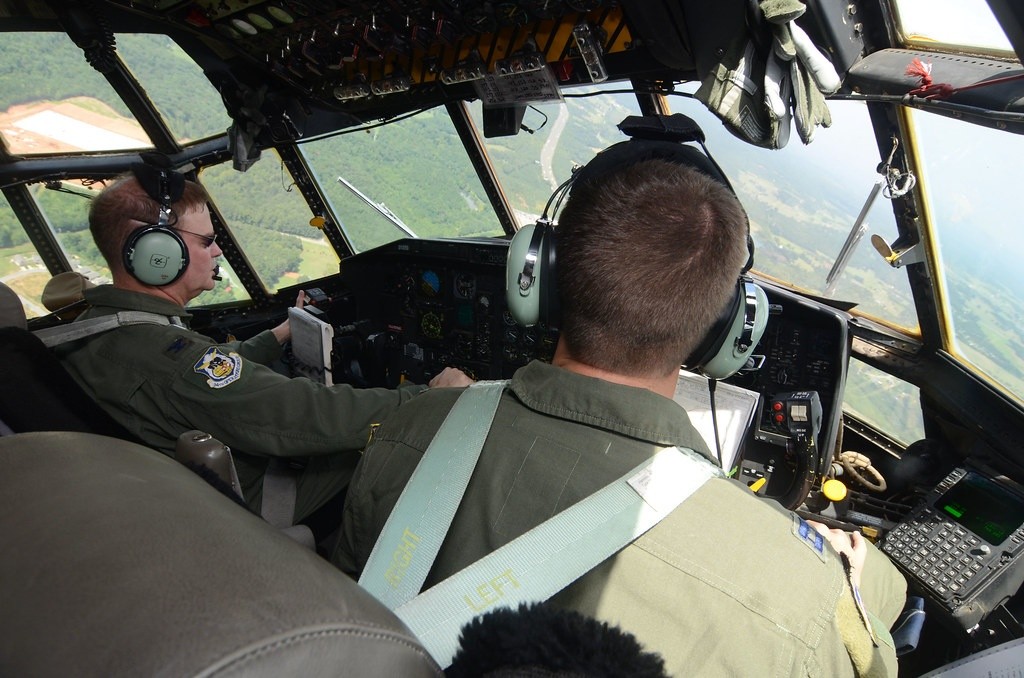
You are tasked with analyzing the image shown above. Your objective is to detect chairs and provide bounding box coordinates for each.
[0,281,444,678]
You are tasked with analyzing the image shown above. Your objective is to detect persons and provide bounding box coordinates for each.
[51,170,478,534]
[327,158,908,678]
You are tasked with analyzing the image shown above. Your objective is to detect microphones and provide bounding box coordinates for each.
[212,265,222,281]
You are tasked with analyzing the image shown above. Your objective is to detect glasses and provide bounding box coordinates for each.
[113,214,217,248]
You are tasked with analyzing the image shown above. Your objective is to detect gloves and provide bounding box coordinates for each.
[227,82,276,172]
[693,0,841,149]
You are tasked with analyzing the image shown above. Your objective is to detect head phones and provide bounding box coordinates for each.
[505,113,770,381]
[122,164,190,286]
